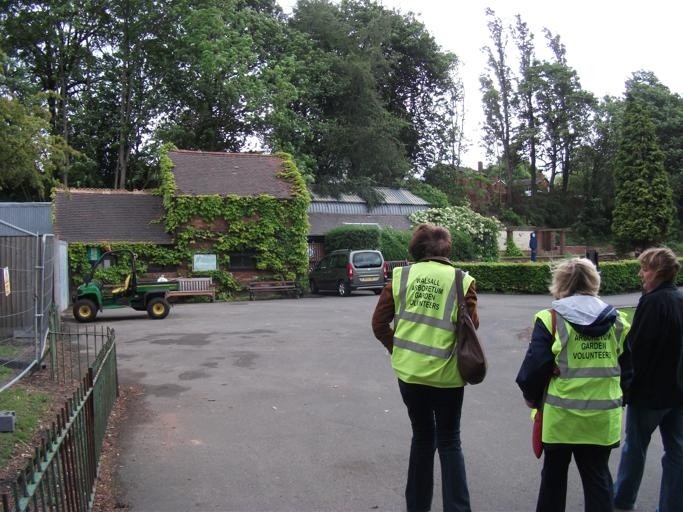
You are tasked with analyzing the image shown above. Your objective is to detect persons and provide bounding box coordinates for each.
[514,257,635,512]
[371,224,481,512]
[610,248,681,510]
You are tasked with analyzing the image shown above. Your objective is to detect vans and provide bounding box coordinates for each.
[308,248,387,295]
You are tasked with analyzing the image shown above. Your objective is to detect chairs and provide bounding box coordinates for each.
[111,274,131,300]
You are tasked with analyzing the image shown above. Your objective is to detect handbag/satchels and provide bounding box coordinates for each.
[456,308,486,386]
[532,409,543,458]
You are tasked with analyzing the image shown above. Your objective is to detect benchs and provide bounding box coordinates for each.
[172,277,215,303]
[249,280,296,300]
